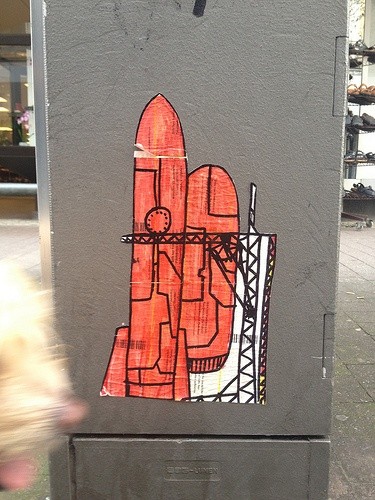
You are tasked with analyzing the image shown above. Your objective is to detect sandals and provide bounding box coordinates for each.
[363,113,375,126]
[366,152,375,162]
[368,85,375,96]
[354,40,368,51]
[351,114,364,127]
[359,84,373,95]
[351,183,375,196]
[355,150,368,162]
[344,150,355,162]
[347,84,359,95]
